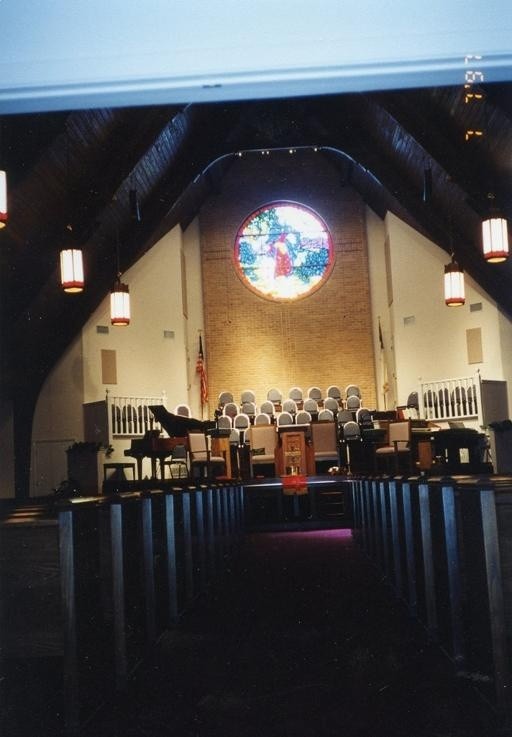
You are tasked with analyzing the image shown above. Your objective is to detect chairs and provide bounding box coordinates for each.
[108,402,192,434]
[160,442,191,482]
[211,381,477,480]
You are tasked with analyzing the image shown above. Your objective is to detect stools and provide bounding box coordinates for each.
[103,461,138,488]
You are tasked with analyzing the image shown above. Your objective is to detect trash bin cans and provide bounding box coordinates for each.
[419,439,432,477]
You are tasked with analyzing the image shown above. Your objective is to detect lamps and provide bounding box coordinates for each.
[1,162,136,332]
[439,166,511,311]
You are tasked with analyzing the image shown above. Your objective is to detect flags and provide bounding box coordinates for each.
[195,333,207,405]
[378,320,390,394]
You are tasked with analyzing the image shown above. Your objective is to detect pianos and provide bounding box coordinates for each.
[123,405,215,481]
